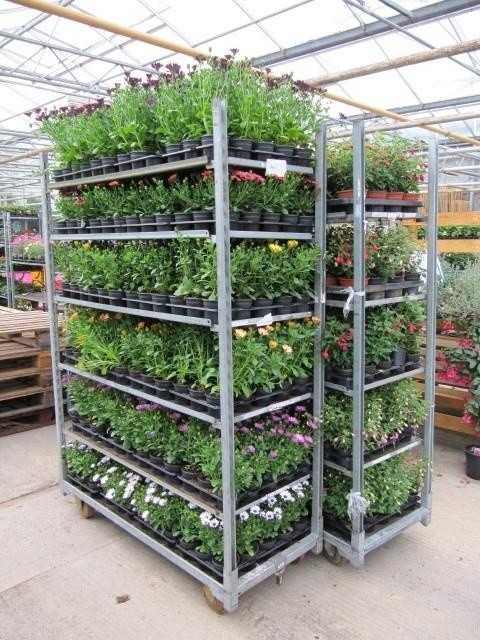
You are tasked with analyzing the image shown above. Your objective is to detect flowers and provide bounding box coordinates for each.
[408,148,428,183]
[63,302,175,344]
[439,305,479,456]
[265,239,299,255]
[406,319,429,337]
[230,171,266,185]
[234,406,317,459]
[56,237,96,255]
[72,195,88,207]
[108,181,121,188]
[62,441,223,536]
[67,374,216,442]
[238,479,308,526]
[236,317,319,354]
[23,46,329,128]
[322,332,353,359]
[334,240,380,269]
[380,425,420,449]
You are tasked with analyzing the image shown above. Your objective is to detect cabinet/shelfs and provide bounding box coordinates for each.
[0,306,64,437]
[401,212,480,438]
[1,204,46,310]
[40,98,328,612]
[319,119,439,569]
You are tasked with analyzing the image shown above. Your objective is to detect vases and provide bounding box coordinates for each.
[464,446,479,481]
